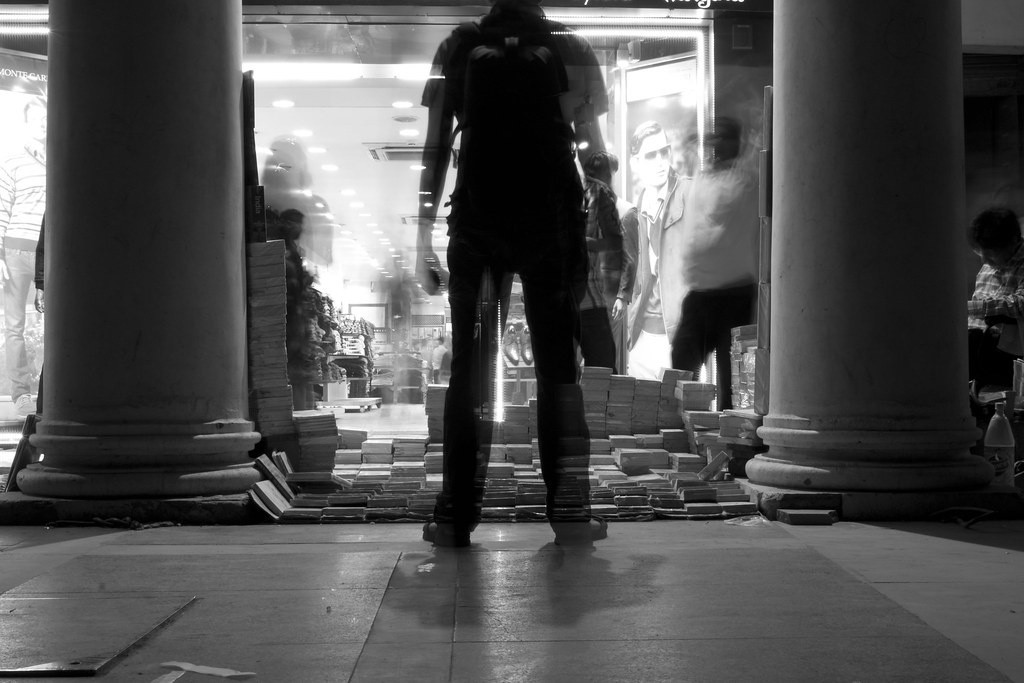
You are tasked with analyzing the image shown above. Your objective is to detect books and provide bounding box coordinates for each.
[244,239,772,522]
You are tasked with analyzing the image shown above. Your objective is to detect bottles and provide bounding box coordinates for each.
[983,402,1015,491]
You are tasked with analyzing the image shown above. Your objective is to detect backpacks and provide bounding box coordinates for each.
[457,24,571,234]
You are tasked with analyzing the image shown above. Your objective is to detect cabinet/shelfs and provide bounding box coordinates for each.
[325,354,383,412]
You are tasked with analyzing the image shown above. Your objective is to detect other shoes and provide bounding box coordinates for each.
[423,518,471,547]
[555,515,607,545]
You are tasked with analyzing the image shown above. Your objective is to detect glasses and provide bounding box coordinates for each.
[639,144,672,162]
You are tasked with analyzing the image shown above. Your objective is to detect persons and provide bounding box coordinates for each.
[34,212,45,415]
[671,118,759,411]
[966,204,1024,392]
[414,0,639,545]
[627,122,706,383]
[575,150,639,377]
[0,97,45,416]
